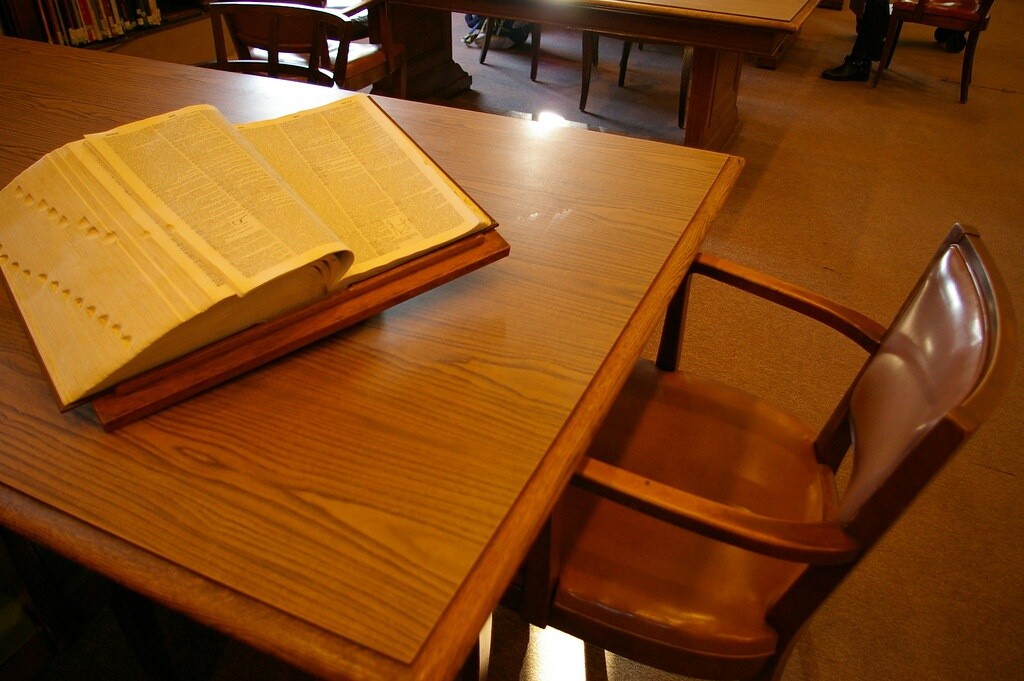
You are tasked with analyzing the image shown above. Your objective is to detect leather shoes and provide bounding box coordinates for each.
[822,62,871,81]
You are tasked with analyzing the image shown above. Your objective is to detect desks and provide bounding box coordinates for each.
[369,0,819,151]
[0,32,746,681]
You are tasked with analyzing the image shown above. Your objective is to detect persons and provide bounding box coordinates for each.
[822,0,889,81]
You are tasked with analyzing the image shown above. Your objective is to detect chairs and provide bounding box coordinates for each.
[481,220,1019,681]
[871,0,996,104]
[191,0,389,95]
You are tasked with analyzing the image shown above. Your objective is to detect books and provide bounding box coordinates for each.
[1,94,498,413]
[12,0,162,46]
